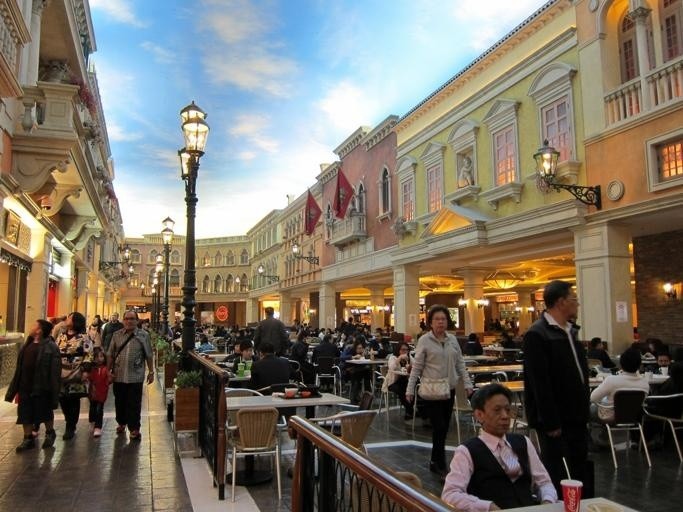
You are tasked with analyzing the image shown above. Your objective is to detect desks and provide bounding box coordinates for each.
[219,393,351,486]
[462,342,526,429]
[490,498,633,511]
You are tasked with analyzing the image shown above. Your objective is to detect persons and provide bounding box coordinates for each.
[458,156,474,187]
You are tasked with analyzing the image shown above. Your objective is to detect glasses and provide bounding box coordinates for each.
[565,296,579,302]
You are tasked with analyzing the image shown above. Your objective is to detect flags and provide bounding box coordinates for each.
[333,167,354,219]
[304,189,322,234]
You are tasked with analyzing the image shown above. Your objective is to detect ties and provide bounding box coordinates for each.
[498,440,520,482]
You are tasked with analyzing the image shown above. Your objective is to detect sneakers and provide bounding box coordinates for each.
[31,430,39,437]
[16,438,35,453]
[130,429,141,440]
[93,427,101,437]
[116,424,126,433]
[430,461,448,476]
[63,427,74,438]
[89,422,95,433]
[43,430,56,448]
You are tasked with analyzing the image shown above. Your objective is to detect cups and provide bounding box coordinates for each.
[659,366,667,376]
[238,363,244,377]
[561,479,584,512]
[400,364,408,372]
[610,368,618,374]
[644,367,653,379]
[245,360,251,369]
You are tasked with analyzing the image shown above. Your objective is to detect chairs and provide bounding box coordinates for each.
[197,327,277,431]
[286,330,462,458]
[223,408,282,502]
[589,351,682,470]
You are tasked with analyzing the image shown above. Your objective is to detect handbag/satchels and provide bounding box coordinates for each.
[418,376,451,401]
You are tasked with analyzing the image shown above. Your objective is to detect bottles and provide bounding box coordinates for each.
[362,347,374,359]
[0,315,5,336]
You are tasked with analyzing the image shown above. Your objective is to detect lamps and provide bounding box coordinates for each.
[532,137,602,212]
[107,264,134,281]
[257,264,280,283]
[291,240,319,265]
[99,243,132,273]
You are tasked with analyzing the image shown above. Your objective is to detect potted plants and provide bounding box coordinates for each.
[148,328,203,430]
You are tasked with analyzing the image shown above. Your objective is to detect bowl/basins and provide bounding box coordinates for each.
[274,384,320,397]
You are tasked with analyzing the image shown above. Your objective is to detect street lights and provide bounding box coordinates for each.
[176,98,210,373]
[150,254,163,334]
[159,216,177,336]
